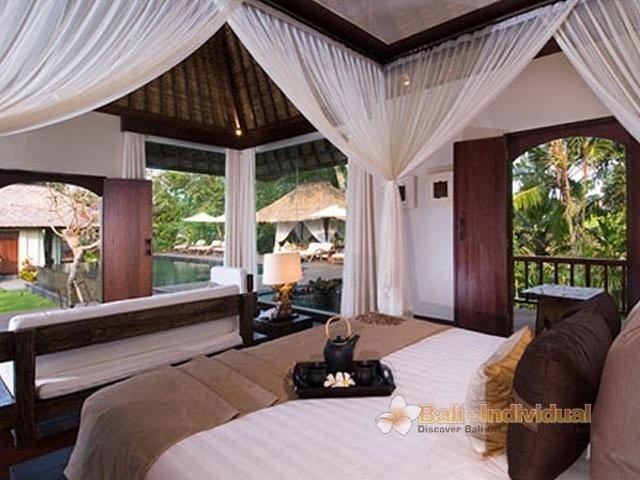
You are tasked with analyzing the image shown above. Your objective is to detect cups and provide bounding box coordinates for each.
[353,359,377,382]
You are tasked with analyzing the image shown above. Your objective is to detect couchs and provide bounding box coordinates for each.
[0,282,255,479]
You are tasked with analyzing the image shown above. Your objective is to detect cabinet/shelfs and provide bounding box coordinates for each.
[518,283,606,335]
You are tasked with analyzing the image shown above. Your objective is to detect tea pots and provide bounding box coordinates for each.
[322,313,361,374]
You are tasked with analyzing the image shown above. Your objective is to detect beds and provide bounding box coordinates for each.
[64,315,590,480]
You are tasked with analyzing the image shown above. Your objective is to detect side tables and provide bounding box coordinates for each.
[253,307,316,341]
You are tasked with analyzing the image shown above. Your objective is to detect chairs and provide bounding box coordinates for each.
[154,267,260,317]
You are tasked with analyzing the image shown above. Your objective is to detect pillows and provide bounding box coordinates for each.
[464,324,535,462]
[589,303,640,480]
[507,290,623,479]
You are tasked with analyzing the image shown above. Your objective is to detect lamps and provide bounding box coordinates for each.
[261,251,302,321]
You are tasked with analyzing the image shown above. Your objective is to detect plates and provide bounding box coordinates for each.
[354,374,384,387]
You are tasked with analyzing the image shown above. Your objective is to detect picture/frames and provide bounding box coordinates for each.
[398,175,417,211]
[423,171,452,209]
